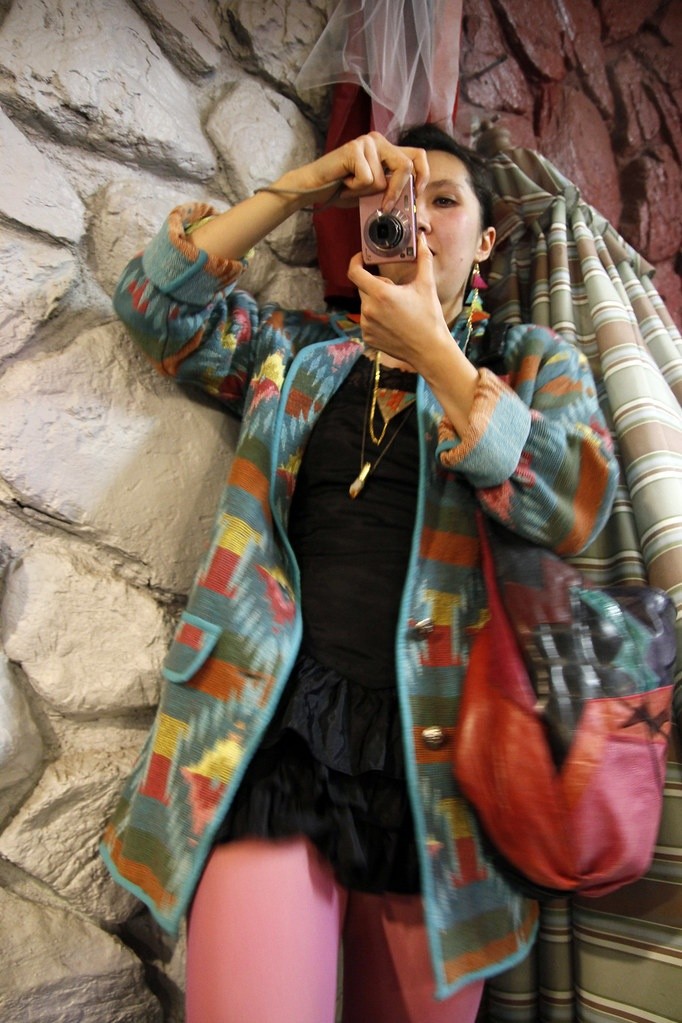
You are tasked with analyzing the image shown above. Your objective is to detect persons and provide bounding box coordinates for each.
[100,122,621,1023]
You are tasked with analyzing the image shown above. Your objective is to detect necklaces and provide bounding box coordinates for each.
[349,284,480,498]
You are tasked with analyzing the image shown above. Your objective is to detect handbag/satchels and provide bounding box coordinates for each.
[451,507,678,900]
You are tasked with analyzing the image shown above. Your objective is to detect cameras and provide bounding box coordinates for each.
[360,174,419,263]
